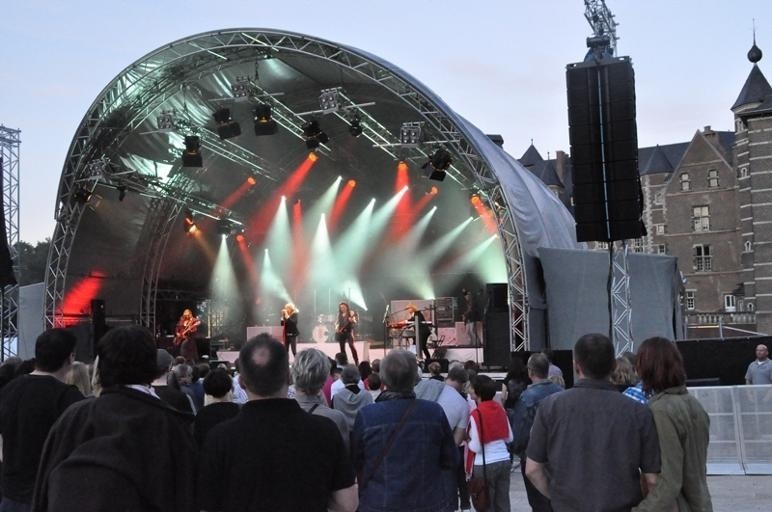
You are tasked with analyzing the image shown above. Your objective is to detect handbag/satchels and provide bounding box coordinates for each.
[468,473,491,512]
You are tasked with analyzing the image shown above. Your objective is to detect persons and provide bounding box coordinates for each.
[744,343,772,386]
[0,326,714,512]
[336,301,362,366]
[401,303,433,358]
[458,288,479,344]
[280,304,301,356]
[173,307,200,362]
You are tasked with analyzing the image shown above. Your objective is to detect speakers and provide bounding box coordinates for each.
[91,300,105,325]
[483,283,510,367]
[566,57,647,242]
[0,157,17,287]
[508,350,574,389]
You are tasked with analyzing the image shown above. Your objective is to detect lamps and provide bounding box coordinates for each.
[74,186,243,244]
[173,92,452,183]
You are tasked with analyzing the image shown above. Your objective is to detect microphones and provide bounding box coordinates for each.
[386,304,389,312]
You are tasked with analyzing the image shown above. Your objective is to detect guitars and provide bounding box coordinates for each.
[172,320,200,347]
